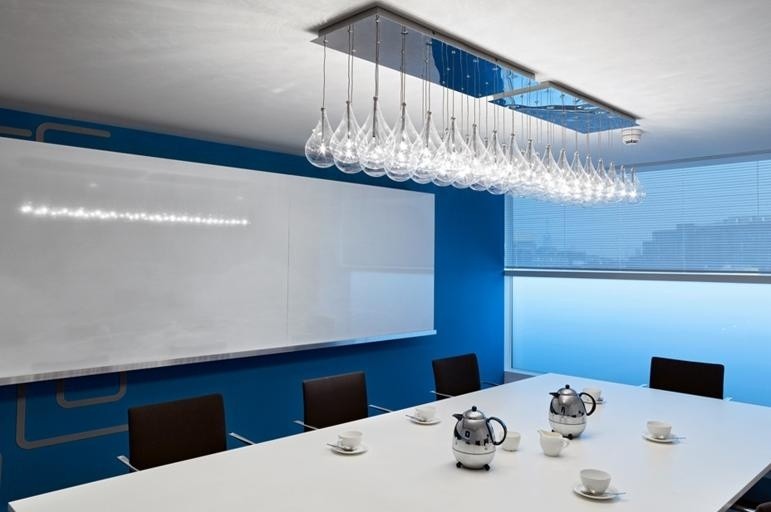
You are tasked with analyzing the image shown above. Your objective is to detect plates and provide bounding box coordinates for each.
[643,431,679,442]
[574,482,618,499]
[332,440,370,454]
[410,415,443,425]
[581,392,605,403]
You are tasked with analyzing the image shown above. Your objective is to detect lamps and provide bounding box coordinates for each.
[305,5,544,197]
[489,80,645,204]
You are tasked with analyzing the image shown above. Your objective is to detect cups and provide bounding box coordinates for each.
[583,387,602,400]
[338,428,363,449]
[646,420,673,437]
[501,431,522,450]
[580,468,612,492]
[415,404,437,422]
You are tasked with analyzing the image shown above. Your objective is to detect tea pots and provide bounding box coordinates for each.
[536,427,571,457]
[451,405,507,471]
[548,384,597,439]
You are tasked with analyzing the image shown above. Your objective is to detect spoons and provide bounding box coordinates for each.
[652,435,688,439]
[405,413,427,421]
[328,442,355,451]
[582,487,627,497]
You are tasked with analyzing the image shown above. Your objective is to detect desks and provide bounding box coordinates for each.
[3,363,771,512]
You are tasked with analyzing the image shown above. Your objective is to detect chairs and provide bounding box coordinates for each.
[639,355,736,402]
[431,353,499,400]
[293,368,394,434]
[115,388,259,473]
